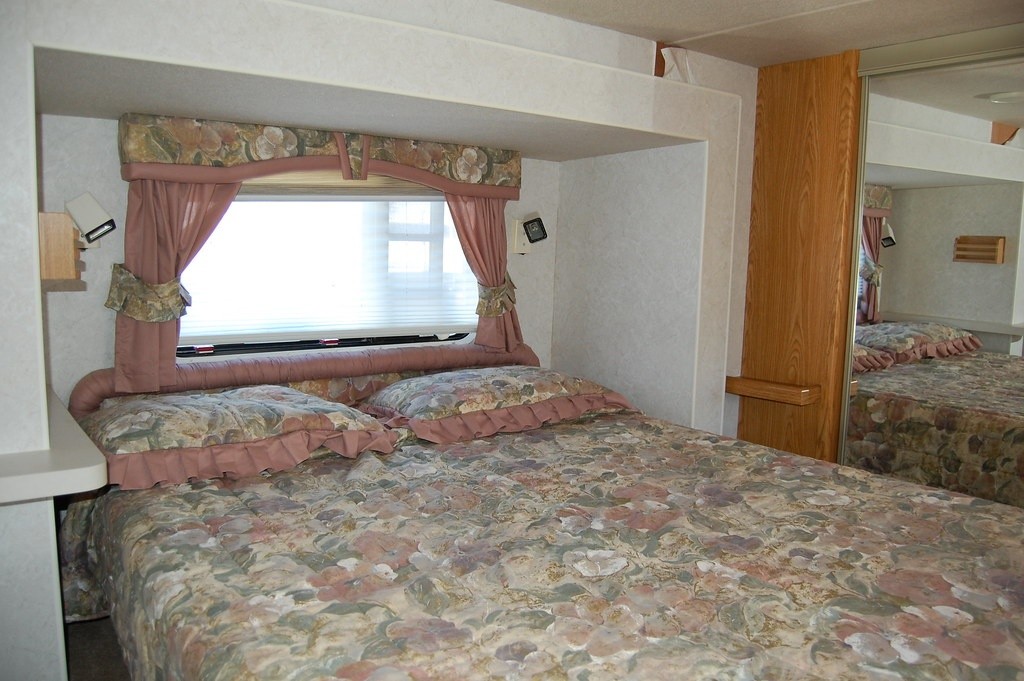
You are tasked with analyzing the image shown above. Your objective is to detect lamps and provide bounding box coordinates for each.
[512,211,548,253]
[880,217,896,248]
[64,190,117,249]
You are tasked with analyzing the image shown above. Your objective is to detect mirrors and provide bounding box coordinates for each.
[836,46,1024,508]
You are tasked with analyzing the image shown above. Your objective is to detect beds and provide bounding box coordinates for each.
[57,343,1024,681]
[844,310,1024,508]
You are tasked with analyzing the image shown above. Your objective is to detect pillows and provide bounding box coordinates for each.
[75,384,399,491]
[357,365,632,445]
[855,321,984,364]
[852,343,894,375]
